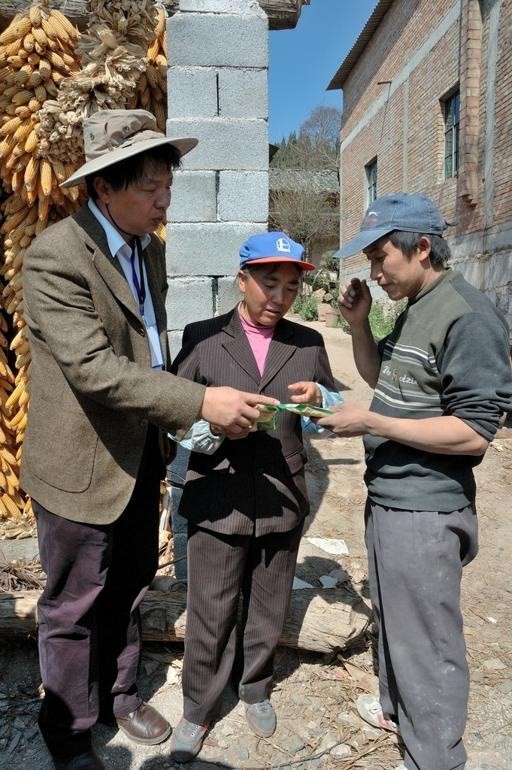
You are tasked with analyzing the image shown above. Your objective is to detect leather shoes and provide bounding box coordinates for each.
[95,700,173,746]
[52,746,107,770]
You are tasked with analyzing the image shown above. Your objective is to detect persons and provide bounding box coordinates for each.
[20,104,283,770]
[159,233,337,762]
[308,186,510,769]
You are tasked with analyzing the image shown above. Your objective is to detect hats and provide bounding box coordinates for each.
[236,231,316,274]
[57,106,201,190]
[331,189,450,261]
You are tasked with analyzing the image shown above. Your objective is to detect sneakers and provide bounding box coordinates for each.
[356,693,405,738]
[394,763,409,770]
[243,698,277,739]
[169,715,212,764]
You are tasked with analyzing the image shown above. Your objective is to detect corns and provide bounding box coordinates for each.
[0,0,167,521]
